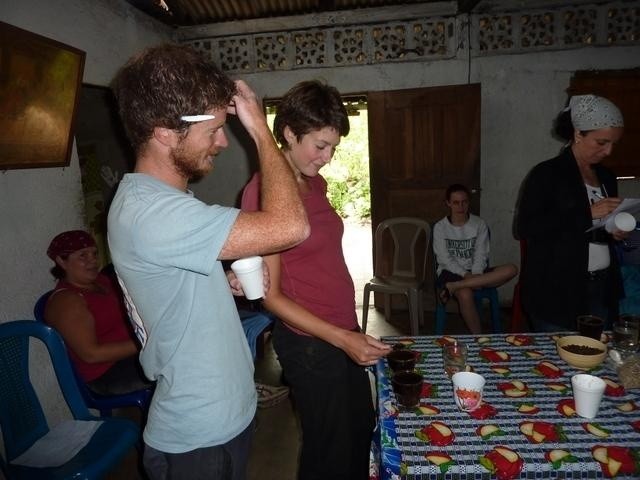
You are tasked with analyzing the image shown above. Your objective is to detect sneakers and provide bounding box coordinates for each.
[254,381,290,408]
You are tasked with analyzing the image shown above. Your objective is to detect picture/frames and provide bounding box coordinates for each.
[0,21,86,170]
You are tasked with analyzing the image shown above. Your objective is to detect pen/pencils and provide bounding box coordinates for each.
[592,190,605,200]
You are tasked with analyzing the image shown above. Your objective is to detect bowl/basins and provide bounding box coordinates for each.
[555,334,607,369]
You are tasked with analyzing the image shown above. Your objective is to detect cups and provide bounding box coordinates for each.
[611,312,639,354]
[442,341,467,374]
[230,255,266,301]
[386,349,417,372]
[390,370,424,408]
[450,371,489,411]
[576,313,605,340]
[572,374,607,419]
[607,211,637,234]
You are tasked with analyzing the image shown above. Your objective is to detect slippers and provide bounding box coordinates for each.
[439,287,450,304]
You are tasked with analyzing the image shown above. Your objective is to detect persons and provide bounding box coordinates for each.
[43,229,154,394]
[431,184,518,333]
[241,77,388,479]
[109,45,311,479]
[512,95,633,332]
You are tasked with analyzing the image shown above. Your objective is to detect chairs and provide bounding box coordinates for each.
[510,240,526,333]
[0,320,148,479]
[362,216,431,336]
[434,227,502,335]
[34,290,154,432]
